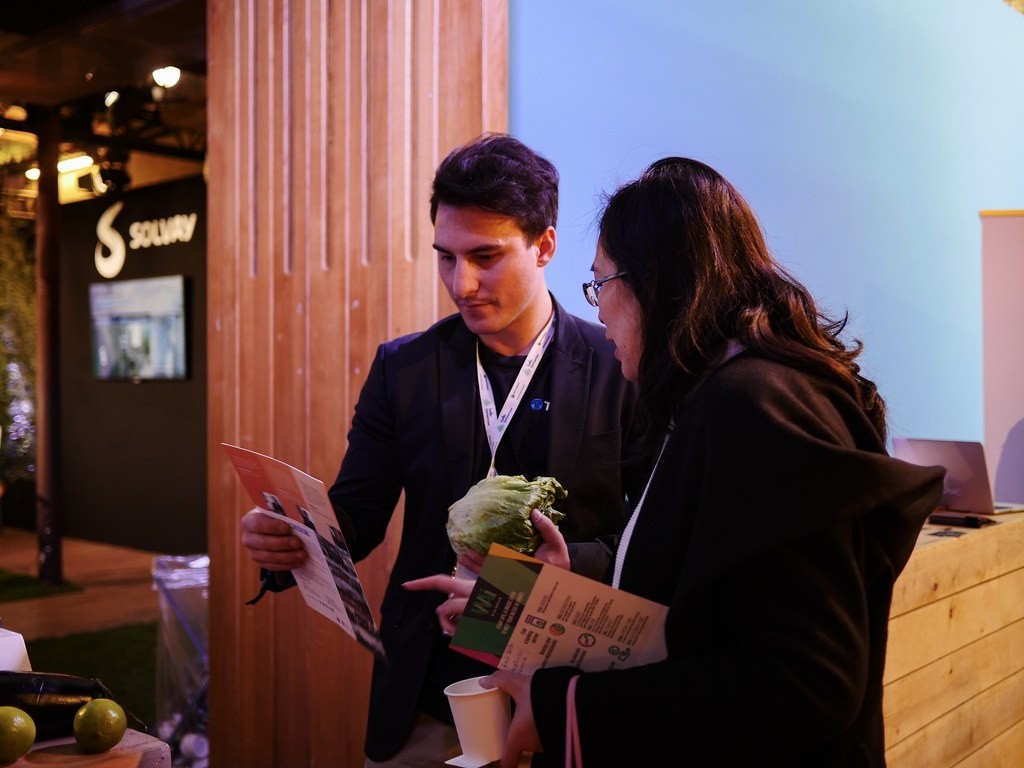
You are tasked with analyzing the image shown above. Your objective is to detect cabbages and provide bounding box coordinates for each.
[446,475,568,555]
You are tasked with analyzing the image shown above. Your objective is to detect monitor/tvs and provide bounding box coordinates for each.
[88,274,193,384]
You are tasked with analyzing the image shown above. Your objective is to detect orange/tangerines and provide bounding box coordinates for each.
[0,706,36,765]
[74,699,127,750]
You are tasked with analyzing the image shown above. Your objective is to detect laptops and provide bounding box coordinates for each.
[892,438,1024,516]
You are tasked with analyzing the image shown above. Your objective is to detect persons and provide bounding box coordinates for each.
[402,156,948,768]
[242,131,623,768]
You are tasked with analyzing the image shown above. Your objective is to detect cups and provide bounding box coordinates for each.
[444,675,512,762]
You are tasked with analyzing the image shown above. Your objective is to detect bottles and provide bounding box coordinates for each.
[150,553,210,768]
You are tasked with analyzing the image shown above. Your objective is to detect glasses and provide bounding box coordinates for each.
[582,271,630,308]
